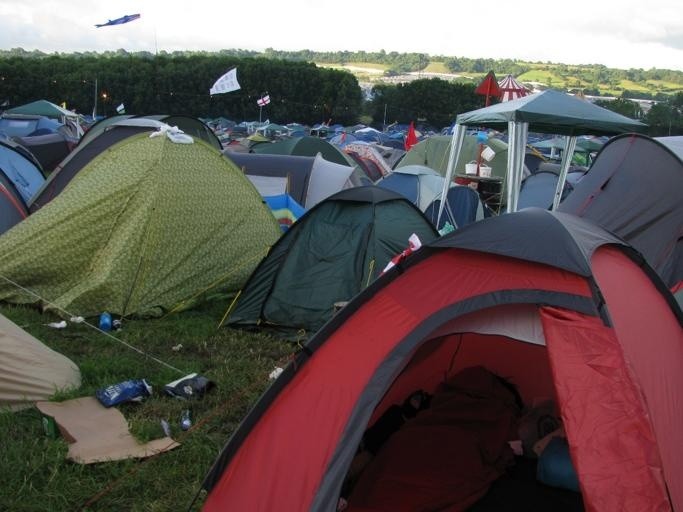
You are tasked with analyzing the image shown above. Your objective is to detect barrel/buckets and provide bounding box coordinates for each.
[479,163,492,177]
[466,161,478,175]
[481,145,496,162]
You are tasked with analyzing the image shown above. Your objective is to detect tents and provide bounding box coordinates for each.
[0,316,81,410]
[187,205,682,510]
[1,100,682,349]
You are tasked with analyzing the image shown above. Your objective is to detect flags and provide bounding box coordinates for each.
[208,67,241,94]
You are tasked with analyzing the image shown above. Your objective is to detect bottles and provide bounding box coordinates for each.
[99,311,112,330]
